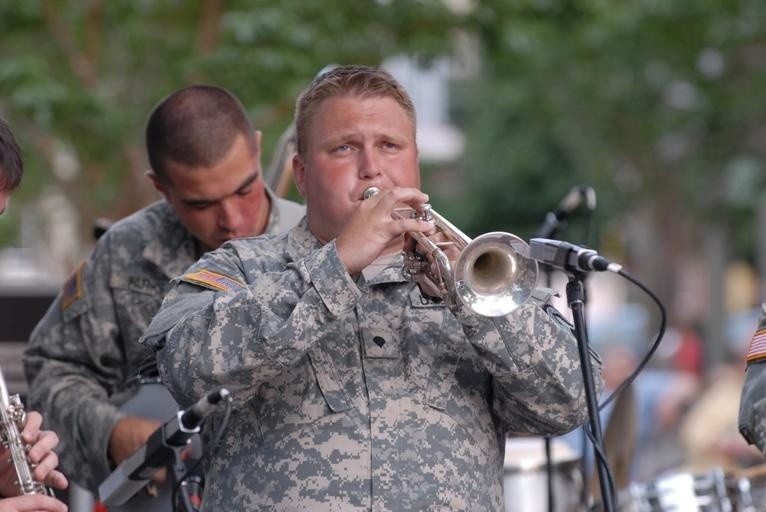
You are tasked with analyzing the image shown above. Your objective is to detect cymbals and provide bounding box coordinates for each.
[591,382,636,498]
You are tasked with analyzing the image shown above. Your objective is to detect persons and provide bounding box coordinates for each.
[0,118,69,511]
[138,63,604,511]
[737,300,766,452]
[19,85,307,511]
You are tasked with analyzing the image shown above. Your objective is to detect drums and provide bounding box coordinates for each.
[504,437,586,511]
[593,467,758,512]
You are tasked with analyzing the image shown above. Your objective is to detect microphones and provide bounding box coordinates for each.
[183,459,206,512]
[528,238,619,273]
[96,386,227,504]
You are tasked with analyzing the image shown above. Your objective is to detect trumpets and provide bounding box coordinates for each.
[363,186,538,316]
